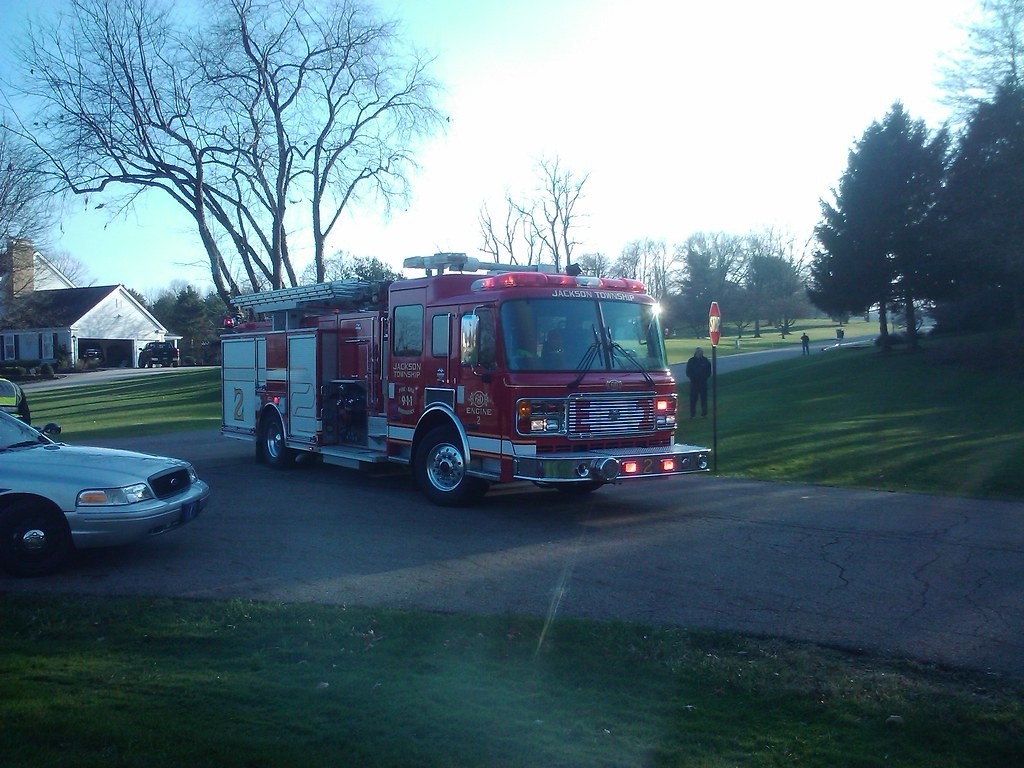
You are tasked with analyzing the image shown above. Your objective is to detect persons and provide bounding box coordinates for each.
[0,360,31,426]
[664,327,669,337]
[686,346,711,420]
[800,333,809,356]
[735,335,741,354]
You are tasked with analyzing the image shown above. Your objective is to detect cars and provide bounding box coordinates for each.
[0,410,210,579]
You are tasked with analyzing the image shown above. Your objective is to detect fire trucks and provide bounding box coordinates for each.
[219,253,712,508]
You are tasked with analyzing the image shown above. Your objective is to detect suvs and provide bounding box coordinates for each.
[79,343,106,367]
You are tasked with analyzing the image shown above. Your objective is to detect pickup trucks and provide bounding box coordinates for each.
[138,342,179,368]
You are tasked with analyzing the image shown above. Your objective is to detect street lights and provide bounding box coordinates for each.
[71,335,76,365]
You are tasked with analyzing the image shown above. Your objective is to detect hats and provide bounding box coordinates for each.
[695,346,704,351]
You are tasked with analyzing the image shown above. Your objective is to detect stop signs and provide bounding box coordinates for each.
[709,301,721,346]
